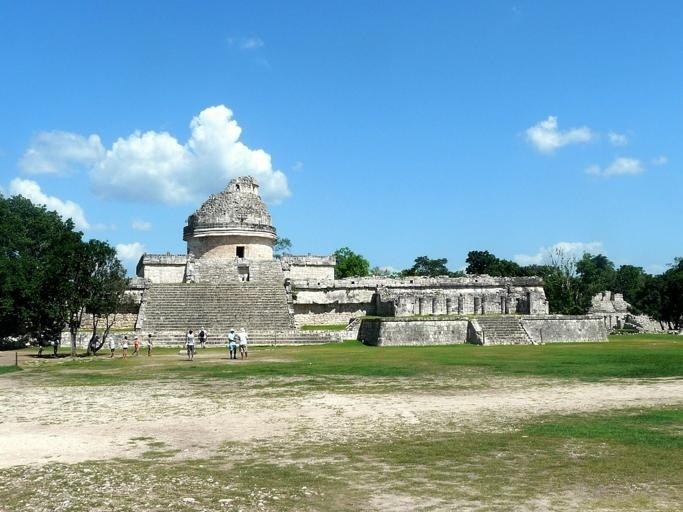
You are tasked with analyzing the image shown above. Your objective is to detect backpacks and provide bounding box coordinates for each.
[200,330,204,337]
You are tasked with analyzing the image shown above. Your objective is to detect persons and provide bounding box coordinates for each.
[227,328,237,359]
[237,327,248,360]
[107,337,115,358]
[91,335,100,355]
[197,326,207,348]
[184,329,194,361]
[120,335,128,360]
[146,334,153,356]
[130,337,140,357]
